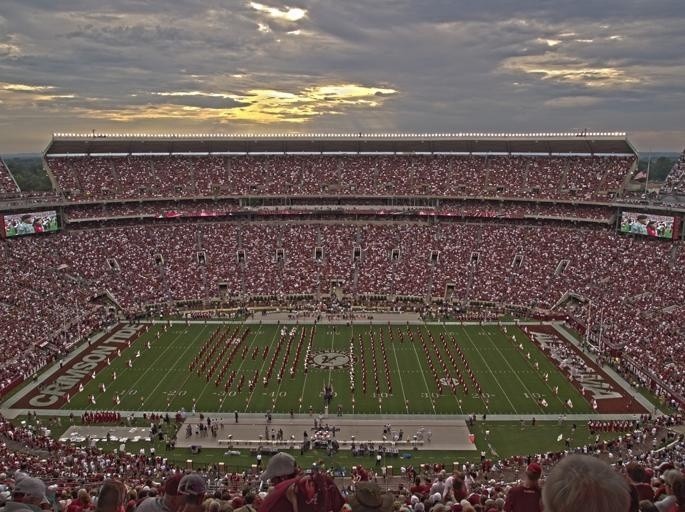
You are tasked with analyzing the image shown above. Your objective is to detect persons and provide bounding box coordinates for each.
[1,146,685,511]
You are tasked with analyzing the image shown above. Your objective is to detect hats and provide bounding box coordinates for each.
[527,464,542,480]
[14,478,50,504]
[166,473,205,496]
[350,482,394,512]
[261,451,296,480]
